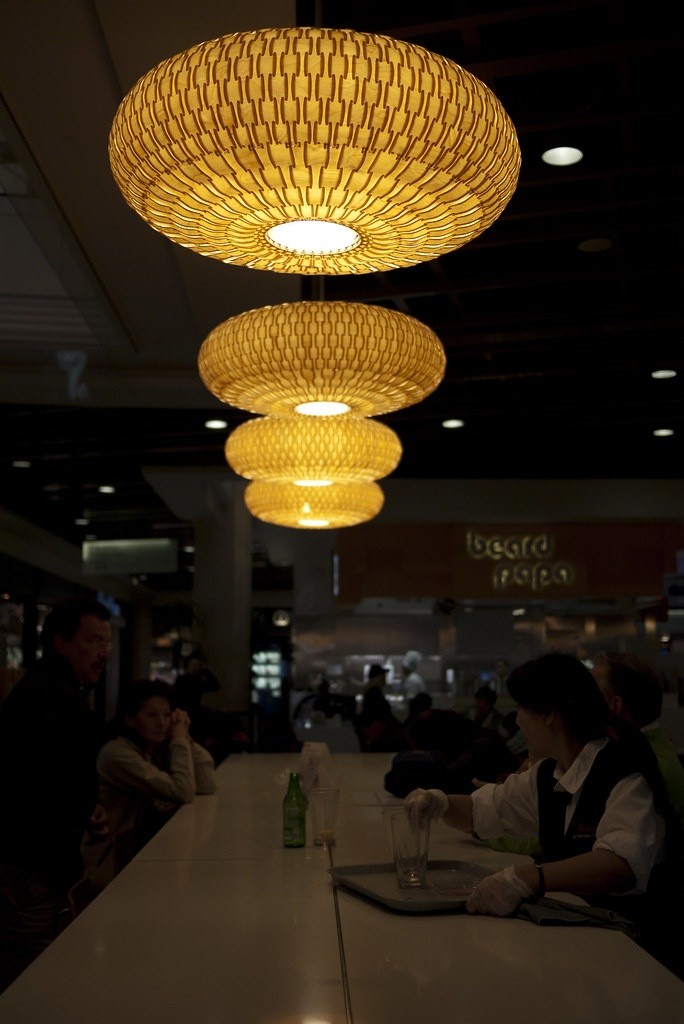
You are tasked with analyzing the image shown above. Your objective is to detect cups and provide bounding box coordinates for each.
[391,811,430,891]
[311,786,339,845]
[297,743,329,798]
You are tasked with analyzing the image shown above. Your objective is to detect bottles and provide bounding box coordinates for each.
[282,770,307,847]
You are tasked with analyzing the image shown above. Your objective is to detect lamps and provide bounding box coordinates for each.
[195,300,448,420]
[242,478,386,531]
[224,415,405,487]
[104,26,524,277]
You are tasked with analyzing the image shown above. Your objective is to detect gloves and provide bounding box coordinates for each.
[403,788,448,833]
[466,865,536,919]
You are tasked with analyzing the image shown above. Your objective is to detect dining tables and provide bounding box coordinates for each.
[2,750,683,1024]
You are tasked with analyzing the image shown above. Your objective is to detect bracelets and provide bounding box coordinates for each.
[532,859,547,898]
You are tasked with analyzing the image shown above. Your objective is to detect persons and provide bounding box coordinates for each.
[469,647,683,818]
[399,692,477,752]
[0,595,111,995]
[80,673,217,898]
[170,650,223,757]
[438,648,461,694]
[353,706,410,753]
[473,686,520,745]
[489,656,515,693]
[358,661,403,734]
[451,668,485,720]
[400,648,683,983]
